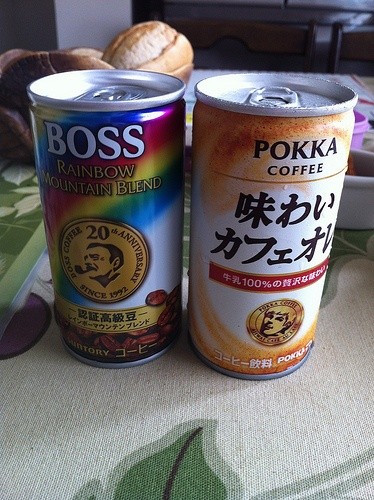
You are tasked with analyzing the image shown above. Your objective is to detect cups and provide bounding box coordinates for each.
[322,148,374,230]
[351,109,369,152]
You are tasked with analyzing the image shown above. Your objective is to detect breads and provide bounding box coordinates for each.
[101,21,194,86]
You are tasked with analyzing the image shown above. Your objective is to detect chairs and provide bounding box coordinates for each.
[326,22,374,76]
[150,17,317,72]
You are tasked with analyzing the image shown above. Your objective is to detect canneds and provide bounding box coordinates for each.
[186,74,357,380]
[28,69,187,366]
